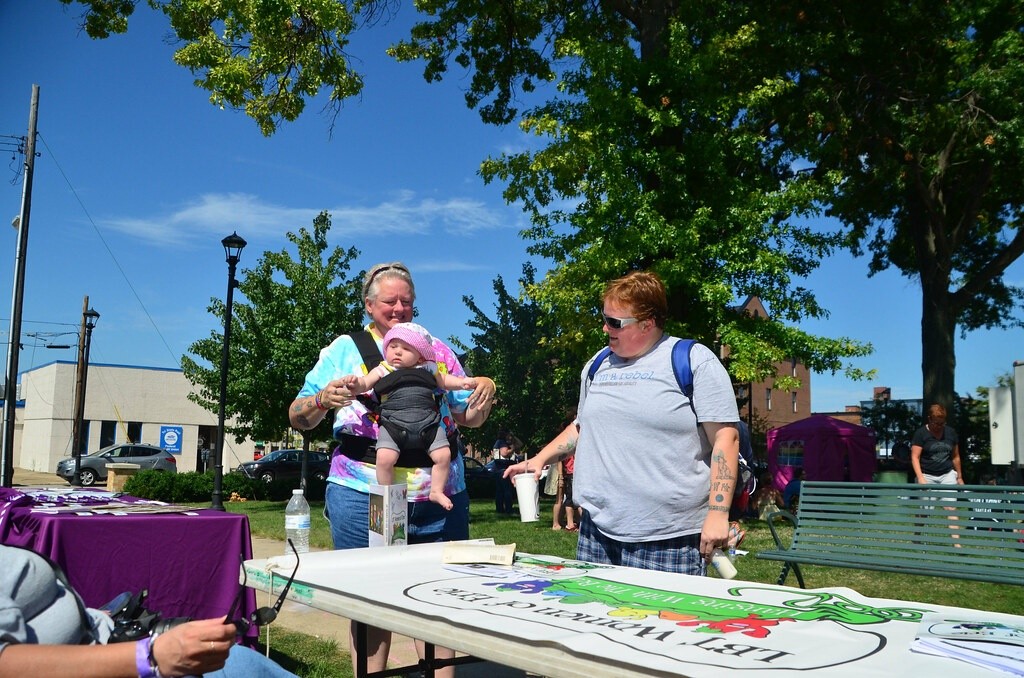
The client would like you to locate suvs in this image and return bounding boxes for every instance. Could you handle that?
[57,442,177,486]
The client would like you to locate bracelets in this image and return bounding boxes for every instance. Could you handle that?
[315,390,329,410]
[148,632,165,678]
[488,378,496,398]
[135,637,155,678]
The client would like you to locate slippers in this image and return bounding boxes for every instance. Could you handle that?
[729,522,739,537]
[736,530,746,549]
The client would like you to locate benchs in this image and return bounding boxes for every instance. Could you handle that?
[747,483,1024,590]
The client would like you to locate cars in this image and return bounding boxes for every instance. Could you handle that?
[463,457,486,498]
[236,450,330,482]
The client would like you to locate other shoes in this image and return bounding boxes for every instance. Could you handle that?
[565,525,578,532]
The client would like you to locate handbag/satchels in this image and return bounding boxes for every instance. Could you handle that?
[544,462,557,495]
[758,500,782,520]
[495,445,517,479]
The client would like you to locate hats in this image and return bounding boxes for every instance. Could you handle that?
[736,395,749,410]
[382,322,435,360]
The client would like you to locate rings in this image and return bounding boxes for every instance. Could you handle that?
[210,640,217,654]
[335,388,337,394]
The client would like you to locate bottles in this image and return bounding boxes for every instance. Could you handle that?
[708,546,738,579]
[729,543,736,564]
[285,489,310,559]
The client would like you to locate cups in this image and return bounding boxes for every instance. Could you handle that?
[514,473,540,523]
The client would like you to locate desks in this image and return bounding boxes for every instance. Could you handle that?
[237,538,1024,678]
[0,487,259,648]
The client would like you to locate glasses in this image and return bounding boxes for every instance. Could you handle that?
[223,538,300,636]
[601,310,653,329]
[930,417,947,427]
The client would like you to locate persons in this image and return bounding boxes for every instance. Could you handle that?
[493,431,516,460]
[783,467,808,508]
[286,262,497,678]
[550,454,583,532]
[0,543,303,678]
[908,401,969,553]
[338,321,479,511]
[752,472,784,522]
[502,271,741,577]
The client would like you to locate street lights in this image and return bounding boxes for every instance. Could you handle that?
[882,389,890,460]
[210,230,248,512]
[69,308,100,486]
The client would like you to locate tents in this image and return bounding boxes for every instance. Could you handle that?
[763,415,877,493]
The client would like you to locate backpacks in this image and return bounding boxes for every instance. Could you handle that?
[589,339,752,497]
